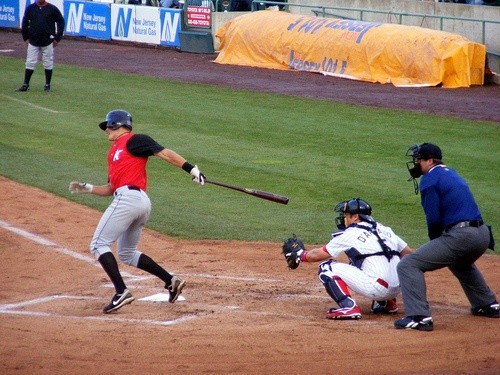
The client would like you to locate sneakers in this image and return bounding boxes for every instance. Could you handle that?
[470,299,500,318]
[324,306,362,320]
[395,316,433,331]
[165,276,186,303]
[44,85,50,93]
[373,300,398,313]
[19,85,27,91]
[102,290,135,313]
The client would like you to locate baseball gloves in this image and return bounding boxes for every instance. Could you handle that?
[283,238,305,270]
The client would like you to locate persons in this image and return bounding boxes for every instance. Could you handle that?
[394,143,500,332]
[283,197,413,320]
[69,110,207,314]
[16,0,65,93]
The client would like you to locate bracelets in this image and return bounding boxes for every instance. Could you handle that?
[182,162,193,174]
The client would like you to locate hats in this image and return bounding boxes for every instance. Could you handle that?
[418,143,444,165]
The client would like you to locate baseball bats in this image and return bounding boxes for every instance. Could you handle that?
[200,179,289,204]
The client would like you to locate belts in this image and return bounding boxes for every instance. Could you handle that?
[115,186,140,196]
[451,220,484,229]
[377,279,388,289]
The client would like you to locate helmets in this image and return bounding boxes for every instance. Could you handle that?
[345,199,376,223]
[98,110,132,132]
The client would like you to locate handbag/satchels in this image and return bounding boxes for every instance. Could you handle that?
[485,225,495,251]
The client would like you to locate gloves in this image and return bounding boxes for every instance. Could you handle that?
[182,163,206,186]
[69,181,94,194]
